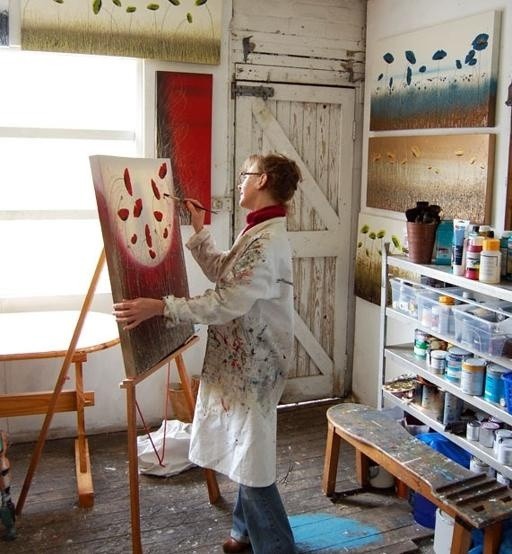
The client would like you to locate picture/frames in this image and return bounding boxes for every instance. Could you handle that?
[351,210,410,312]
[368,8,503,133]
[366,131,498,227]
[19,0,223,67]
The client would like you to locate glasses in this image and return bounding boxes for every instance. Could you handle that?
[239,170,262,183]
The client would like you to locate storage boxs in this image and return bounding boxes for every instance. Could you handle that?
[387,277,424,318]
[415,290,468,338]
[451,303,512,359]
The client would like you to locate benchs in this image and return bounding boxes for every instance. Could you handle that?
[320,401,511,554]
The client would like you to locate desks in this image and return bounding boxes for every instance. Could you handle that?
[0,311,122,510]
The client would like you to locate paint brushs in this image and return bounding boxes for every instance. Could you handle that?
[163,193,218,215]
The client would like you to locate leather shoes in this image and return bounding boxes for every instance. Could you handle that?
[222,534,254,554]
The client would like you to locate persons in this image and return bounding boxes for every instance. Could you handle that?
[112,152,303,554]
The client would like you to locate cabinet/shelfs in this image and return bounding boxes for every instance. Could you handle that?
[376,241,512,494]
